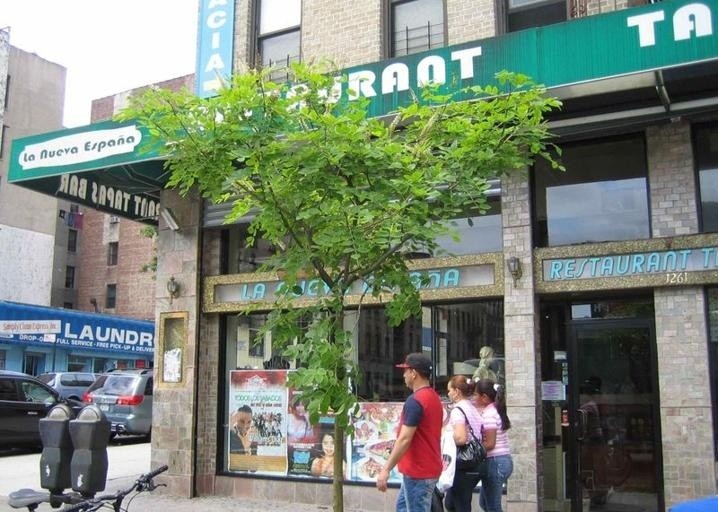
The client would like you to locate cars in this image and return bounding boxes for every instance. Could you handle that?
[0,368,152,448]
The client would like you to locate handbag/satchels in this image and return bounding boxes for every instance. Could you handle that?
[436,425,455,492]
[451,407,486,468]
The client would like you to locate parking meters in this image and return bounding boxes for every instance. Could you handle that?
[38,403,111,500]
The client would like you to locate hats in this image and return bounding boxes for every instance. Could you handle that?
[394,352,434,374]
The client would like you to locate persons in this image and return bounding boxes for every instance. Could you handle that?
[308,430,346,478]
[376,353,445,512]
[228,403,259,474]
[286,394,313,441]
[472,344,501,384]
[473,378,514,511]
[431,376,486,511]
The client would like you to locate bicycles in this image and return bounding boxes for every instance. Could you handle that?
[8,465,169,512]
